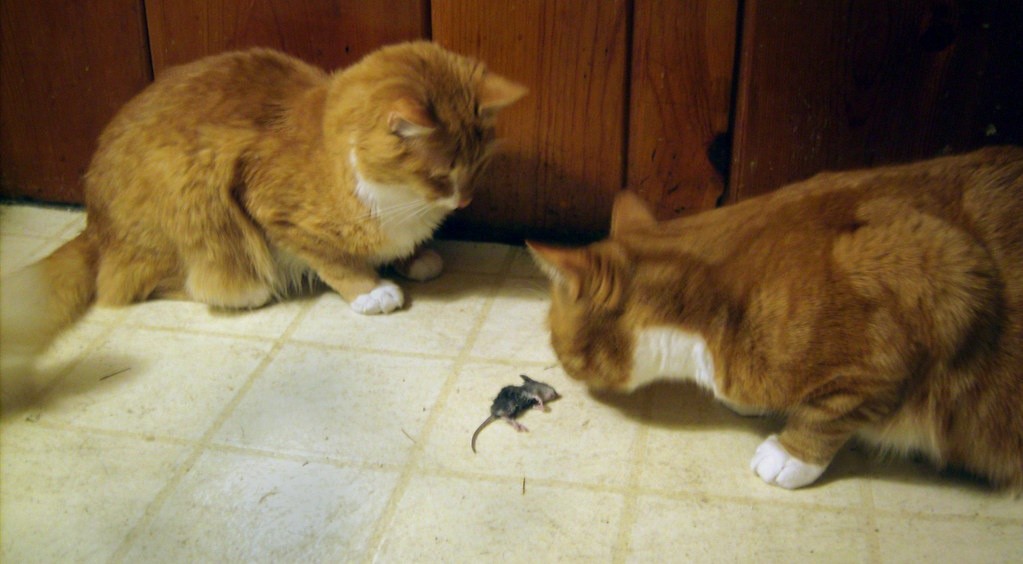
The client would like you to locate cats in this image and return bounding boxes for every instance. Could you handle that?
[525,144,1022,499]
[1,41,527,414]
